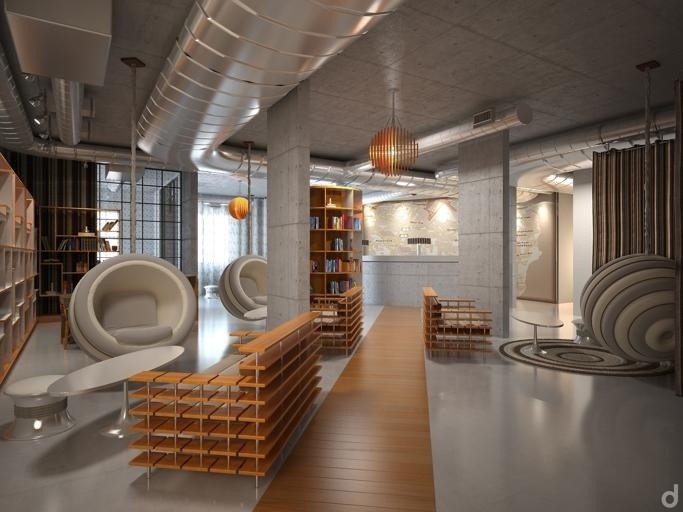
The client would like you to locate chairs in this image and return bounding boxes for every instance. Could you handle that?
[67,256,198,361]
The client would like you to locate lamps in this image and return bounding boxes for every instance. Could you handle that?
[369,89,418,178]
[23,71,51,144]
[228,160,250,220]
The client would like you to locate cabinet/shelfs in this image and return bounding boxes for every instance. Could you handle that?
[0,155,119,383]
[421,286,493,359]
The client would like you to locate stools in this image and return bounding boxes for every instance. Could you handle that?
[1,375,77,443]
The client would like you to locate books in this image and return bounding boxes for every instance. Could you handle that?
[40,220,118,313]
[310,213,361,294]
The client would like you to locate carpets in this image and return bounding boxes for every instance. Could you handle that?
[499,337,674,377]
